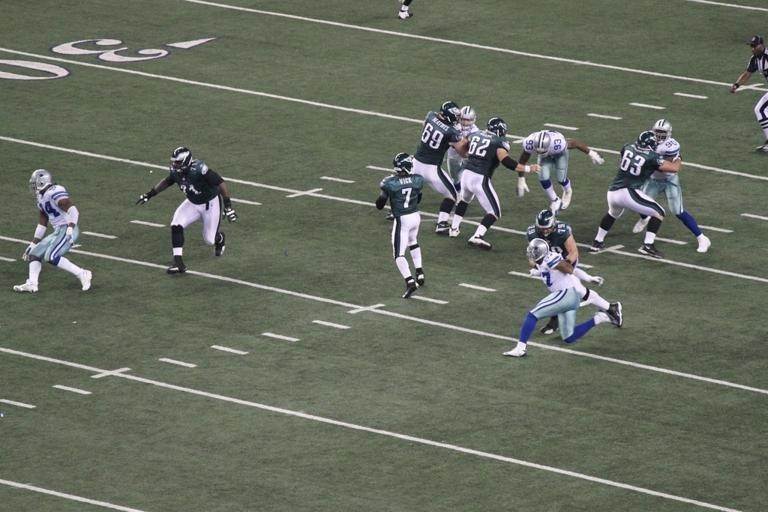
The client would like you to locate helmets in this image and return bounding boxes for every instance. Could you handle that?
[652,119,673,144]
[394,152,415,177]
[531,132,551,155]
[440,101,507,135]
[526,238,549,268]
[634,130,657,152]
[169,147,193,175]
[29,169,52,197]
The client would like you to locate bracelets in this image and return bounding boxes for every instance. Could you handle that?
[734,83,739,88]
[30,242,36,248]
[66,227,73,235]
[223,196,231,208]
[148,189,156,196]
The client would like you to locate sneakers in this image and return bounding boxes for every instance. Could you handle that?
[398,10,414,20]
[79,268,93,291]
[386,209,394,220]
[215,232,226,256]
[503,302,622,357]
[402,282,420,298]
[167,264,186,273]
[548,198,561,216]
[434,220,494,250]
[415,272,424,285]
[13,278,39,293]
[756,143,768,153]
[592,216,711,259]
[560,191,571,209]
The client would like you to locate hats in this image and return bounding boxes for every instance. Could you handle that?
[746,36,765,46]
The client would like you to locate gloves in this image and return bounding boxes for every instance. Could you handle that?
[516,177,530,197]
[457,156,468,170]
[63,226,74,246]
[222,206,238,222]
[587,150,605,166]
[22,241,36,261]
[589,275,604,288]
[528,269,542,279]
[135,191,151,205]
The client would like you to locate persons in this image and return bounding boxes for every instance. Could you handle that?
[13,169,92,293]
[136,147,238,274]
[398,0,413,20]
[729,34,768,153]
